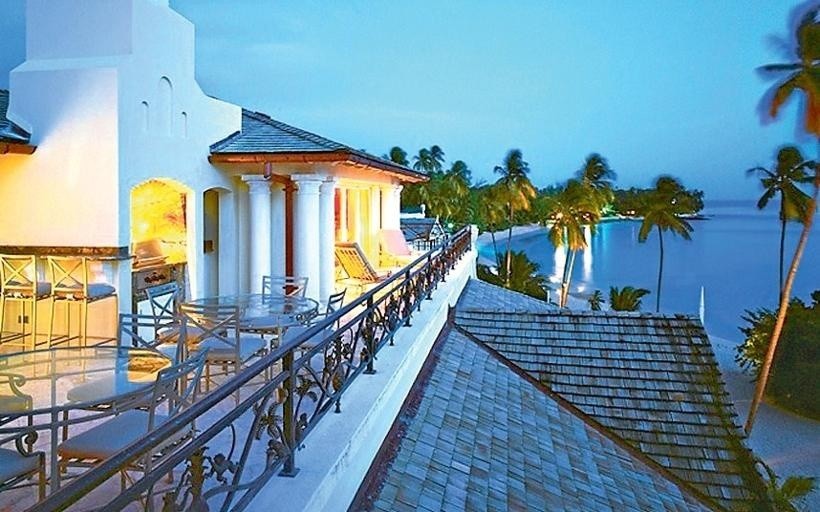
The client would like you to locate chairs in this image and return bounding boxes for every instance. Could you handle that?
[268,286,347,387]
[58,344,212,507]
[263,273,310,298]
[178,301,270,418]
[114,312,188,420]
[336,227,433,309]
[0,355,37,482]
[0,420,48,509]
[144,279,229,394]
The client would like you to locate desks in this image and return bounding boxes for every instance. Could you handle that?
[180,294,320,386]
[1,347,176,492]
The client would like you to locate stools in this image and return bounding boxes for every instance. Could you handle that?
[0,252,71,352]
[46,254,119,351]
